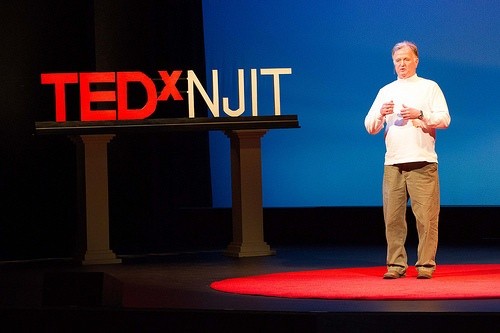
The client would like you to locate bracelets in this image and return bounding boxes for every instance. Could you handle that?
[419,110,423,119]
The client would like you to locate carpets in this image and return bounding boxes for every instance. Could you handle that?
[210,263,500,301]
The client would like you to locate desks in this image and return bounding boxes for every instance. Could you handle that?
[34,114,303,266]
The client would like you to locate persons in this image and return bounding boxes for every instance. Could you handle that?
[364,42,452,279]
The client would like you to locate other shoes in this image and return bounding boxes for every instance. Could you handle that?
[384,271,406,279]
[417,270,432,278]
